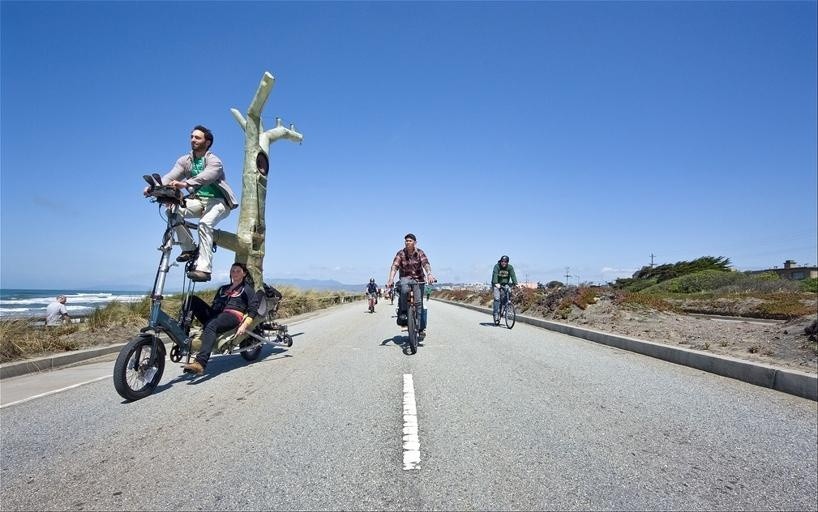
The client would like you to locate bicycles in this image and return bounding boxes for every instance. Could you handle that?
[385,287,397,305]
[385,280,438,354]
[113,172,294,400]
[424,285,435,301]
[366,291,380,313]
[492,282,518,329]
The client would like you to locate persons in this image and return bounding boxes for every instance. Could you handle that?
[177,263,258,377]
[364,276,433,308]
[143,124,234,283]
[44,293,72,328]
[490,254,517,329]
[384,233,434,341]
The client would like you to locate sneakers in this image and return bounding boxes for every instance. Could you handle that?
[187,271,210,281]
[184,362,203,373]
[176,251,194,262]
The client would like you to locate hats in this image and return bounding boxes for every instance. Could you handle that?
[405,234,416,241]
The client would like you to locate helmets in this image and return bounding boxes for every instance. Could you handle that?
[501,256,509,261]
